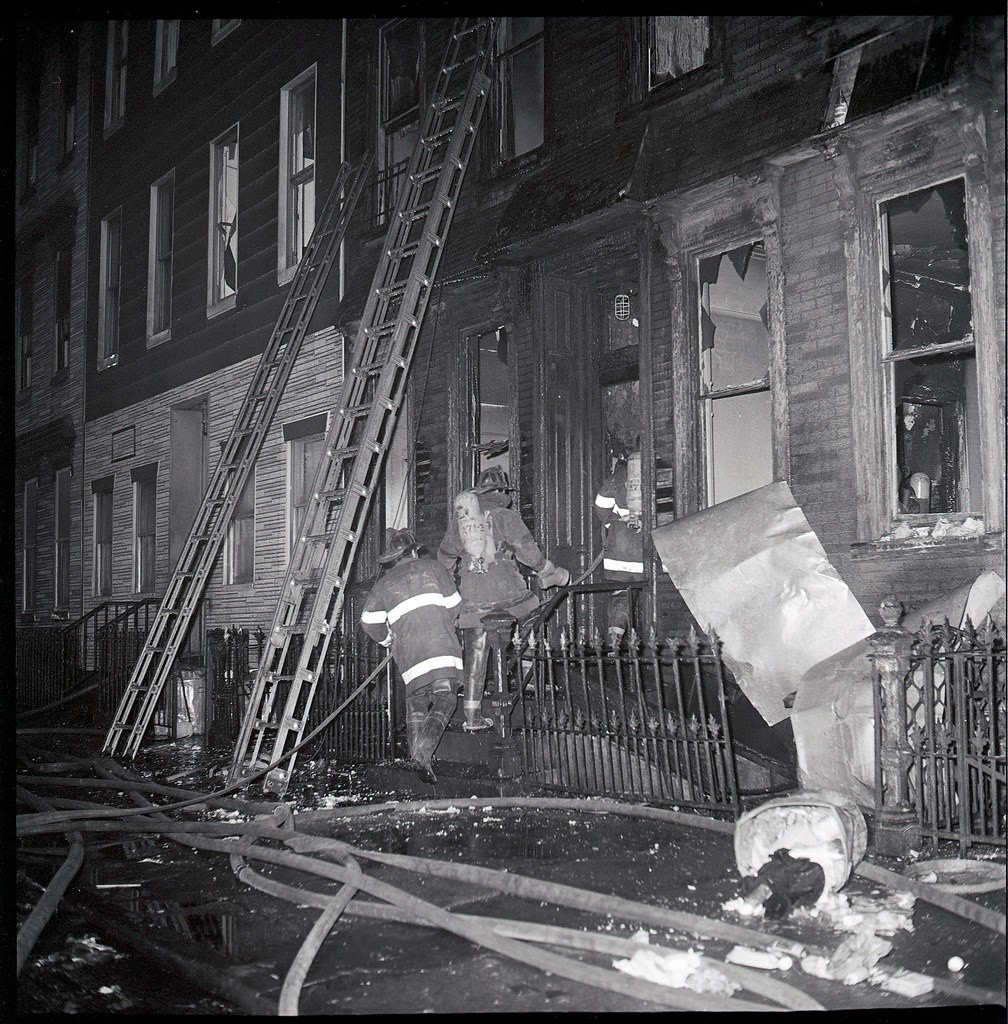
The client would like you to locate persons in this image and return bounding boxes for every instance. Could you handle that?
[437,467,557,728]
[594,435,644,657]
[361,534,465,785]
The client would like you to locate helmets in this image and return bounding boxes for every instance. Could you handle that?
[469,467,515,494]
[377,533,424,562]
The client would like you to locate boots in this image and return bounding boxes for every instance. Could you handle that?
[519,614,557,693]
[406,697,428,758]
[407,692,457,785]
[608,588,631,659]
[463,627,494,733]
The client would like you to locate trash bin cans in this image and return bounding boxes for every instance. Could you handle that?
[154,670,212,738]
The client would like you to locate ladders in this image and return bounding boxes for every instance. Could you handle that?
[230,17,511,796]
[101,147,375,759]
